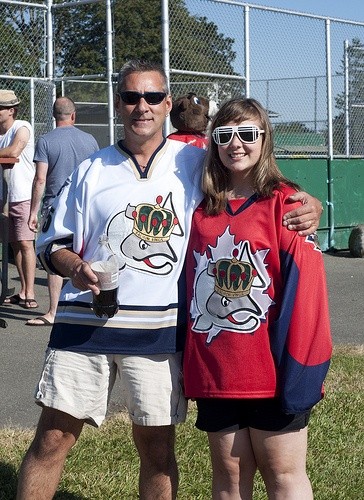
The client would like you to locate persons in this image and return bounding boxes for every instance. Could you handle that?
[175,96,333,500]
[16,60,323,500]
[26,97,100,327]
[0,89,39,308]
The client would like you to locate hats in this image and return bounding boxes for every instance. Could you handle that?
[0,90,21,106]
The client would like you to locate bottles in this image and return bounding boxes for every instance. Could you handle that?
[88,233,119,319]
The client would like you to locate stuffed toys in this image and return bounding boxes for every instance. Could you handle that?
[166,92,221,151]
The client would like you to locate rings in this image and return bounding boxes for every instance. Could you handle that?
[309,221,313,227]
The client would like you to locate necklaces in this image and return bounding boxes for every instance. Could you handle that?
[139,163,146,168]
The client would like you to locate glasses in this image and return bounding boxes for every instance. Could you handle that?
[212,125,265,146]
[117,91,167,105]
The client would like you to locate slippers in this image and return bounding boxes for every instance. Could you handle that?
[27,316,55,326]
[3,293,39,308]
